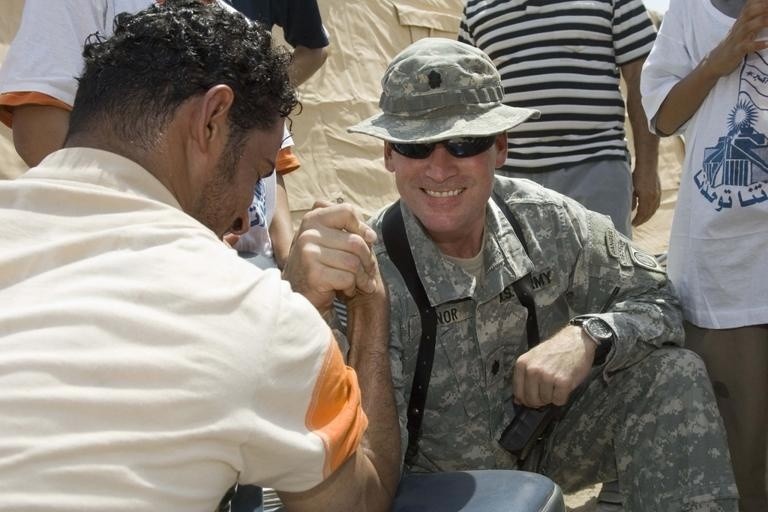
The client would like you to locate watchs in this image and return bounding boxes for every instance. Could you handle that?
[566,315,615,368]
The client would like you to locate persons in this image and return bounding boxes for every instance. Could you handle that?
[2,2,305,512]
[277,35,743,510]
[160,0,333,109]
[1,2,404,510]
[452,2,667,505]
[635,1,768,509]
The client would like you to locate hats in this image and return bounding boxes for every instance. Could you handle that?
[342,34,545,150]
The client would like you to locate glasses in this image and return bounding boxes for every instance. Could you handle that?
[382,131,502,159]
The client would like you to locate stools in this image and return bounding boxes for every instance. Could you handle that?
[390,470,565,512]
